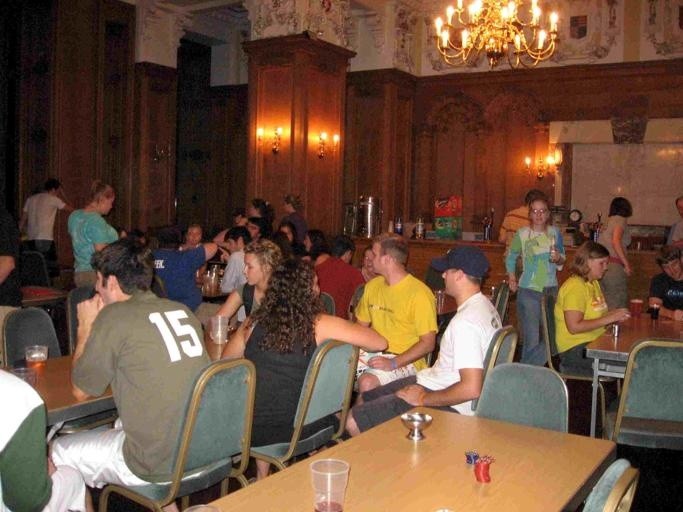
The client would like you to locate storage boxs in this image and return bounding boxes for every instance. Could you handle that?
[462,232,484,241]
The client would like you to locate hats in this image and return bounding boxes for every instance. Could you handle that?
[432,246,490,278]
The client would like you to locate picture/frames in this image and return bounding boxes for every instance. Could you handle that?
[628,224,669,243]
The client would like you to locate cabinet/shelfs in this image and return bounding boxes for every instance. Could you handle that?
[351,239,666,340]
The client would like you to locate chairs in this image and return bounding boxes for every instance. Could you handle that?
[475,364,568,432]
[2,309,115,434]
[470,325,520,411]
[100,358,257,512]
[222,338,359,497]
[65,287,99,354]
[604,339,683,454]
[492,283,510,325]
[18,251,49,289]
[540,287,621,432]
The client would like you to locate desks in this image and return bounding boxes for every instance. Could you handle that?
[21,287,69,323]
[195,406,618,511]
[6,336,248,445]
[586,306,683,437]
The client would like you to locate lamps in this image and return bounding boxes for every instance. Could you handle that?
[428,0,567,68]
[525,156,554,180]
[312,128,340,158]
[257,127,282,153]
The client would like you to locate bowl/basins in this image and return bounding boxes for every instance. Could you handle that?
[399,412,432,441]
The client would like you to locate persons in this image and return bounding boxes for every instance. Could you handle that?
[316,236,368,322]
[22,177,76,276]
[68,180,127,289]
[151,228,237,328]
[246,217,272,240]
[237,200,277,238]
[275,218,310,260]
[648,247,683,322]
[595,197,633,311]
[211,239,283,339]
[667,196,683,251]
[179,226,205,253]
[341,247,502,436]
[0,206,24,369]
[0,369,93,512]
[221,258,388,485]
[351,233,438,406]
[357,245,382,283]
[116,225,154,250]
[554,239,633,426]
[504,197,565,366]
[280,193,308,242]
[301,229,329,266]
[216,226,247,292]
[498,190,549,253]
[45,238,213,512]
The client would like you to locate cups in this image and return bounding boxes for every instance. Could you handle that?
[24,345,48,377]
[9,367,36,392]
[433,290,445,312]
[308,458,350,511]
[629,299,644,318]
[210,314,229,344]
[649,304,660,320]
[200,271,218,295]
[211,265,220,278]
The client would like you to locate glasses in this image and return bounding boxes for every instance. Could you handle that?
[533,209,545,213]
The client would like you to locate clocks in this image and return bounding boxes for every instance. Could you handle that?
[569,209,581,223]
